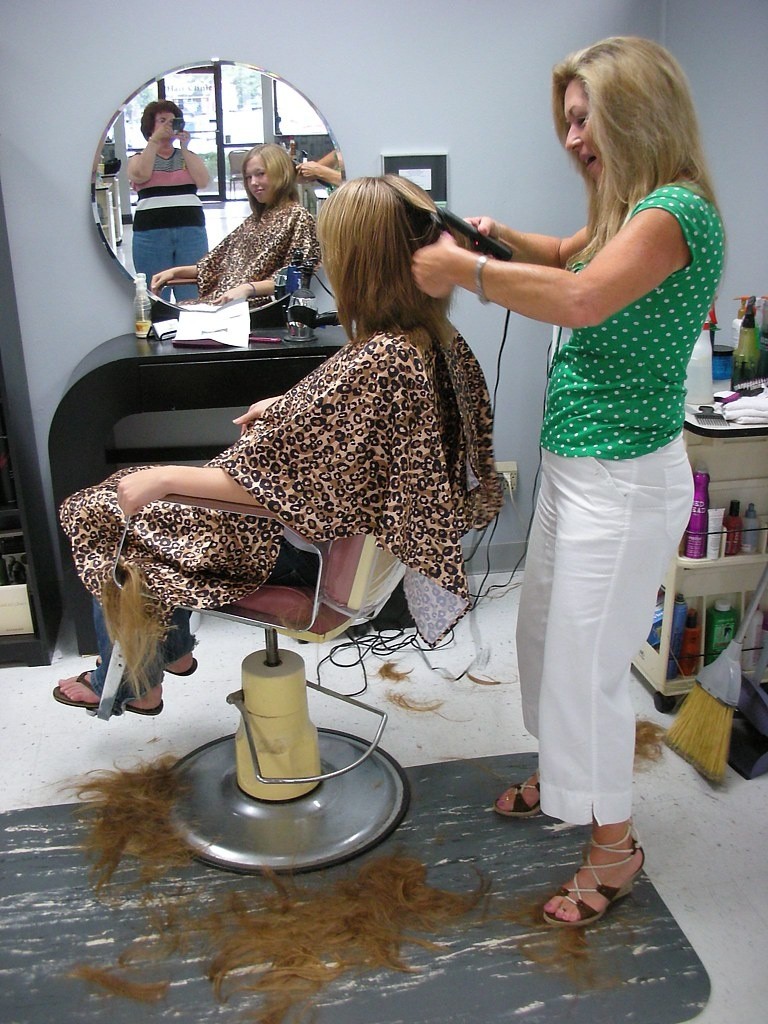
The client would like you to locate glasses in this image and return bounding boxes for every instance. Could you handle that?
[154,118,165,124]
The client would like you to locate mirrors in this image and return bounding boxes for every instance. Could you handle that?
[90,57,346,314]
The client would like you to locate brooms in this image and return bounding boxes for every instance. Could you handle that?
[660,563,768,783]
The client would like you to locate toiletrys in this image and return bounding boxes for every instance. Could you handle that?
[722,499,743,556]
[683,459,712,559]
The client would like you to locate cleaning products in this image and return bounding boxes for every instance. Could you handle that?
[705,598,737,665]
[740,602,765,671]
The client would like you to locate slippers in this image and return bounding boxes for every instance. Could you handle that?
[96,653,198,676]
[54,669,163,716]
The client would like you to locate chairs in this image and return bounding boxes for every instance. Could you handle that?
[87,480,410,878]
[228,149,250,193]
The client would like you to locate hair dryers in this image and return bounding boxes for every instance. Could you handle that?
[272,263,304,301]
[285,287,342,341]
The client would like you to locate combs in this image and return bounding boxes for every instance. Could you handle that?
[720,378,768,401]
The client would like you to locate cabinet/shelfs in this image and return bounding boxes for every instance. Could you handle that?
[630,402,768,714]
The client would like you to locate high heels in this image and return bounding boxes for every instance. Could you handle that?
[543,816,645,928]
[494,769,542,817]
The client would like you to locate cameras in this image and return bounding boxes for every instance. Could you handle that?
[172,117,185,135]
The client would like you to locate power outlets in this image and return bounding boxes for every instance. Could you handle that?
[495,461,517,492]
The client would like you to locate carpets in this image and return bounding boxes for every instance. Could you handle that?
[0,752,712,1024]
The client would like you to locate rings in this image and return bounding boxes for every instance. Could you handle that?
[300,170,302,175]
[166,129,169,131]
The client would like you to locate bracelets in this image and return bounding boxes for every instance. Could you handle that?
[474,257,489,304]
[249,282,258,295]
[148,135,159,143]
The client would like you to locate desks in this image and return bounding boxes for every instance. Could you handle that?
[100,171,123,247]
[48,324,351,658]
[95,182,118,255]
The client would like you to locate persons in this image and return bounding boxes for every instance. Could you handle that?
[128,100,209,301]
[412,36,723,928]
[287,140,304,168]
[151,144,321,309]
[50,174,507,715]
[295,149,343,191]
